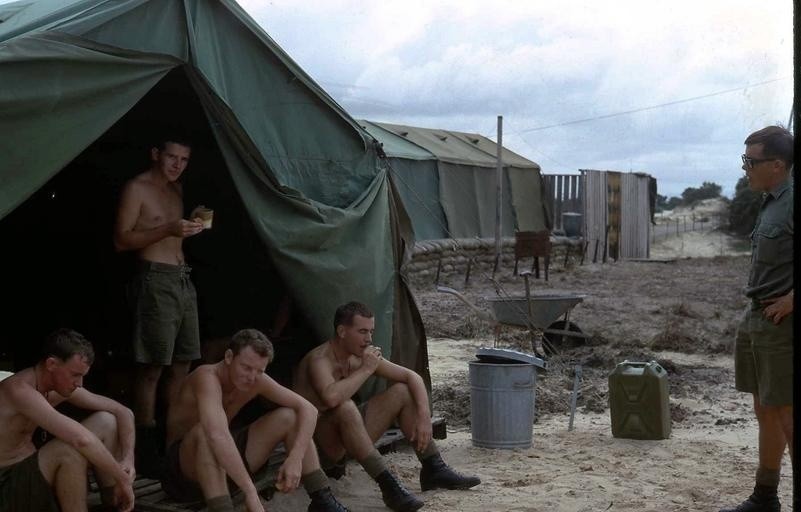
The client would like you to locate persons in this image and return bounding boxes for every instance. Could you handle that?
[0,331,136,512]
[721,126,794,512]
[113,135,203,420]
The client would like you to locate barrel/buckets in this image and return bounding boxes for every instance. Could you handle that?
[607,359,672,439]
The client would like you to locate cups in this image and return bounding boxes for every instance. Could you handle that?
[195,208,215,230]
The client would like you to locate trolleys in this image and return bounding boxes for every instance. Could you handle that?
[437,285,593,362]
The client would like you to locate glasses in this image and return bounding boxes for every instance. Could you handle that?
[742,154,775,169]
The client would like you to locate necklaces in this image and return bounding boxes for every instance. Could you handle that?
[33,366,48,443]
[329,339,351,380]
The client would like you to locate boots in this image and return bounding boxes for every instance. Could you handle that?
[374,469,424,512]
[307,486,351,512]
[420,453,481,491]
[718,483,781,512]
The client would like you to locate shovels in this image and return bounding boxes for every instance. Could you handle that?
[520,269,548,361]
